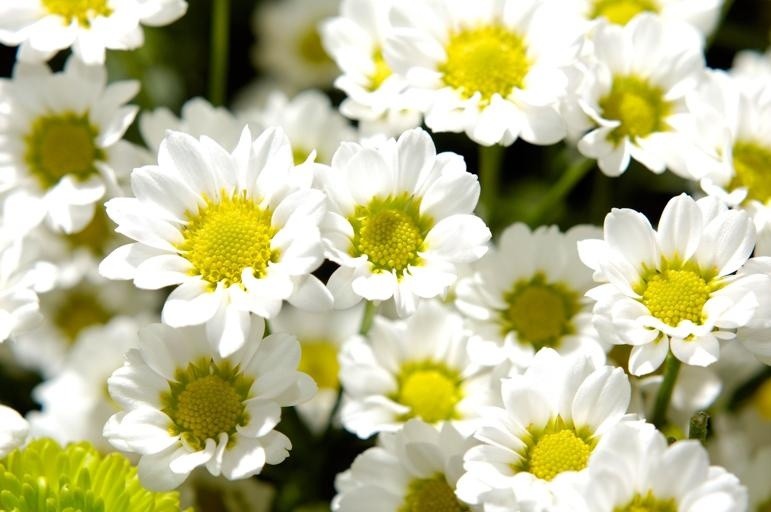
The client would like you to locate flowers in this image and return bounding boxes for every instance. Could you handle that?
[0,0,771,512]
